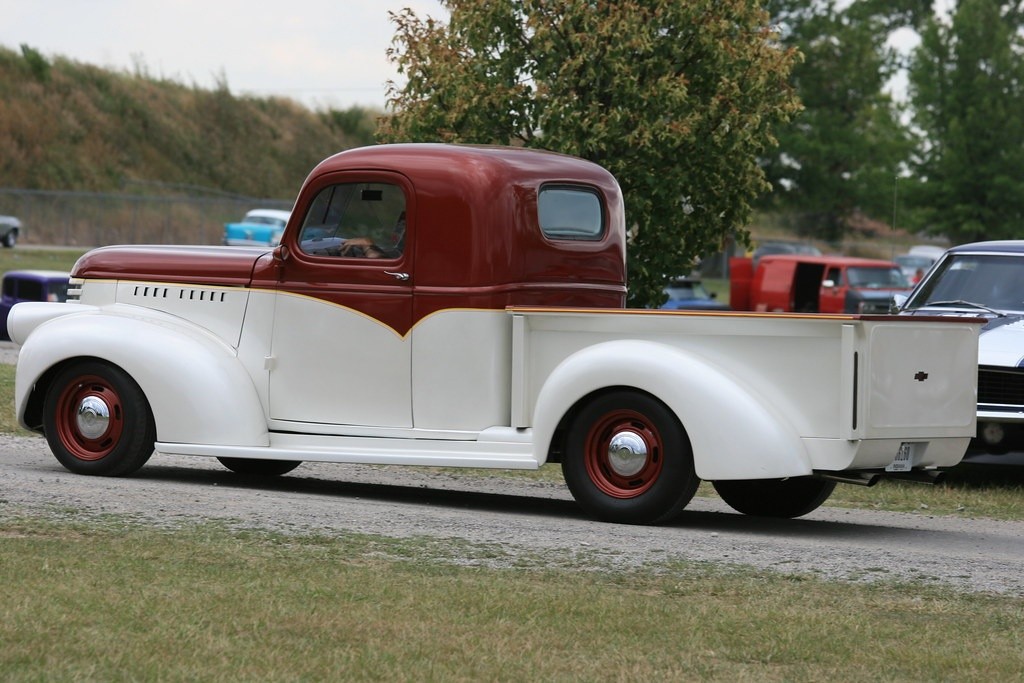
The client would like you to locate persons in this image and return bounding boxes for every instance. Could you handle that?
[912,268,922,284]
[340,238,388,260]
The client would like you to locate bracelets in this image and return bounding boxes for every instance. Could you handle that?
[362,244,378,254]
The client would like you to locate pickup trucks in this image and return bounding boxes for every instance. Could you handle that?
[0,142,989,528]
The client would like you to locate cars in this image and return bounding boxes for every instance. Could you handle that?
[0,215,21,248]
[651,234,1024,459]
[221,206,324,246]
[0,270,73,341]
